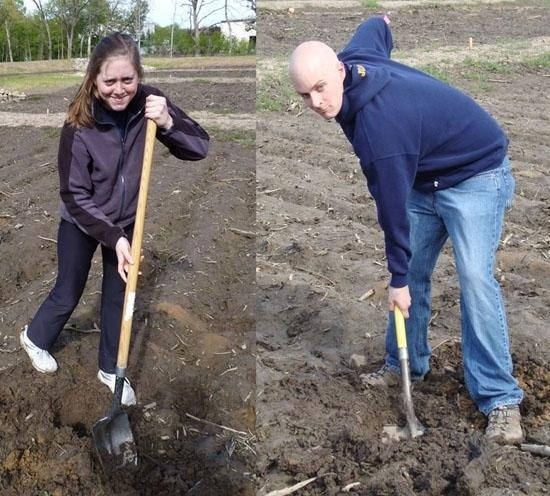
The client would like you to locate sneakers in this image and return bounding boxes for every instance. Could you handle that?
[359,365,424,386]
[20,324,58,373]
[486,404,523,443]
[97,369,137,407]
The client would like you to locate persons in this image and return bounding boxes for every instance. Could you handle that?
[289,15,525,445]
[18,29,210,407]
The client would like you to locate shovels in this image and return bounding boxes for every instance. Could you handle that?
[91,118,156,474]
[375,305,428,442]
